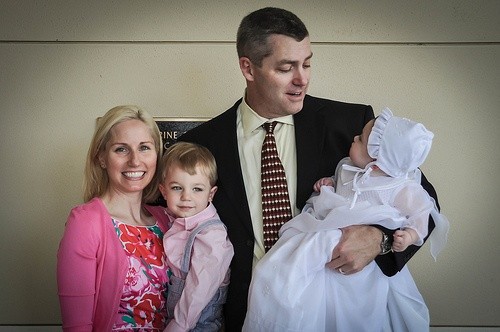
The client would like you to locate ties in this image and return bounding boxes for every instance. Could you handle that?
[259,121,293,253]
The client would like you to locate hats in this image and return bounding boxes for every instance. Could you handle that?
[367,108,435,177]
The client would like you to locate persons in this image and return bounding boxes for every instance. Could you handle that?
[56,104,174,332]
[156,142,235,332]
[178,7,441,332]
[241,108,449,332]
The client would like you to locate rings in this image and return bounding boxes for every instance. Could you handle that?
[339,267,344,273]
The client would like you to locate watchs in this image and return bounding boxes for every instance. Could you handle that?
[381,234,391,252]
